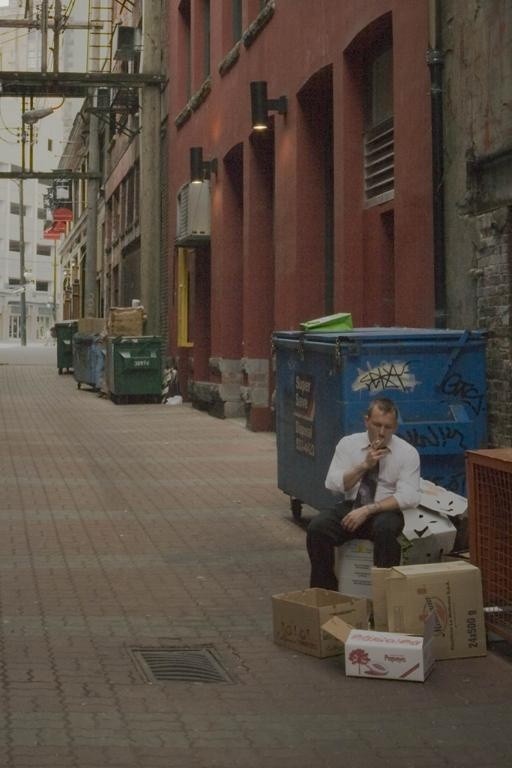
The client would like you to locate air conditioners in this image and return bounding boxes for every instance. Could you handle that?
[174,179,209,247]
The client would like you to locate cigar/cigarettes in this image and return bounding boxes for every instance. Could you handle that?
[375,439,381,444]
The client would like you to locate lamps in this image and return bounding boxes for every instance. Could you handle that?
[190,143,217,185]
[249,77,287,133]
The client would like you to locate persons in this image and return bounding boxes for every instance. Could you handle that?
[304,395,422,592]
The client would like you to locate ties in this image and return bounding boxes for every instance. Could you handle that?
[353,459,380,508]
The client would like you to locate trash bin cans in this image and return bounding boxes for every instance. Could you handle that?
[272,311,489,520]
[55,320,163,404]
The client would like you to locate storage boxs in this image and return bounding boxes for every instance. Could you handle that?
[78,306,146,338]
[272,587,370,658]
[322,616,436,682]
[371,560,487,660]
